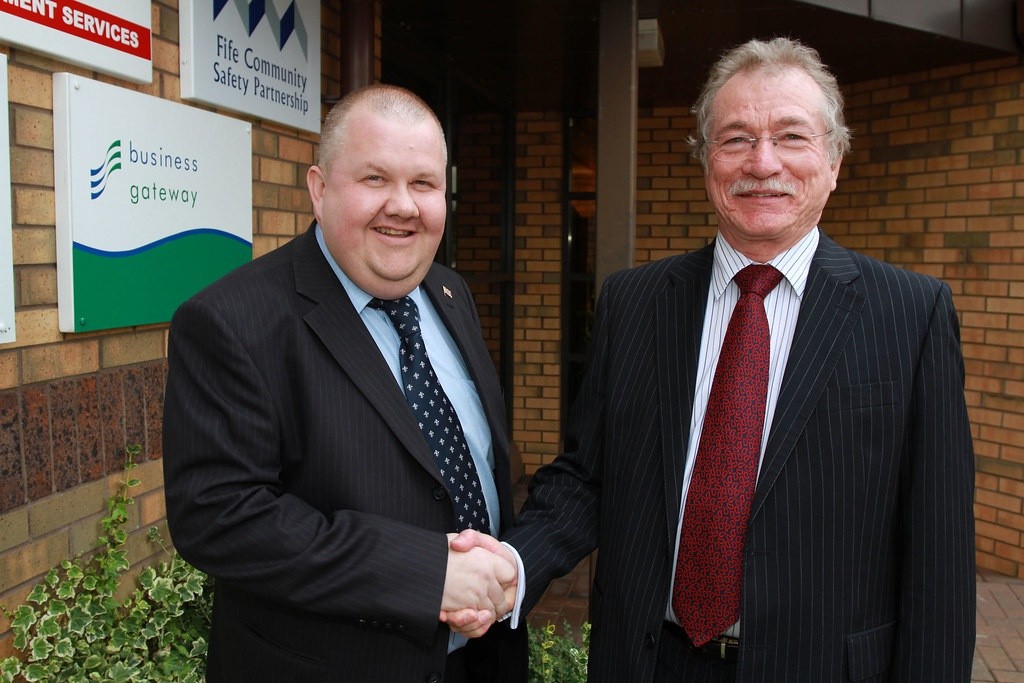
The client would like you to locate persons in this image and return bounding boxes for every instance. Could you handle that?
[161,85,530,683]
[443,37,976,683]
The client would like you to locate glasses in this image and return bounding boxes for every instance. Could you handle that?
[704,128,838,160]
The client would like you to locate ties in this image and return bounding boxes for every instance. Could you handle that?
[670,261,785,647]
[365,299,492,554]
